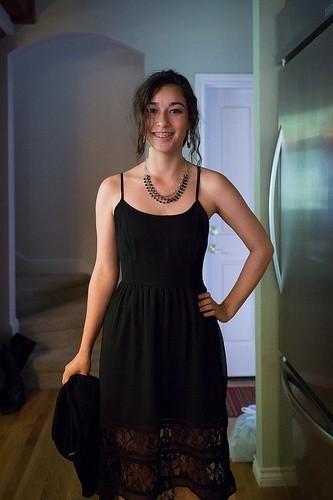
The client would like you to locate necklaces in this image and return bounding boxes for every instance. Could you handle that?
[141,158,190,205]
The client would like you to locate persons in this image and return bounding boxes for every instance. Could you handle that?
[50,69,276,500]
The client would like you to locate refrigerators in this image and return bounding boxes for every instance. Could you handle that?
[268,0,333,500]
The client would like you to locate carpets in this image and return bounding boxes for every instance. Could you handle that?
[225,384,256,417]
